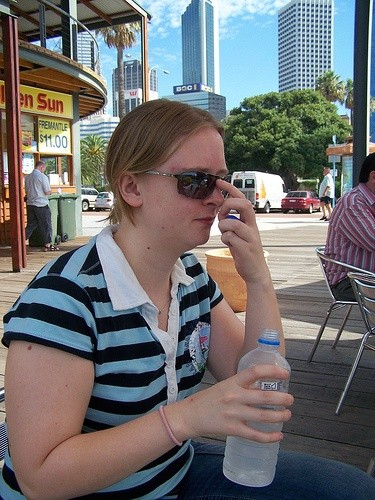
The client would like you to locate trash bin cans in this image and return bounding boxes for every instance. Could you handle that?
[47,199,59,245]
[60,195,78,242]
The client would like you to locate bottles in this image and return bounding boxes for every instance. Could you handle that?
[223,327,291,487]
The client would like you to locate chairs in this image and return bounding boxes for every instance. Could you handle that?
[309,246,375,360]
[335,271,375,417]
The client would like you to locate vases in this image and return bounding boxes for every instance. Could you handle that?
[205,247,268,314]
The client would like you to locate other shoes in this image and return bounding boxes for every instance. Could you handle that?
[320,216,326,220]
[325,218,330,221]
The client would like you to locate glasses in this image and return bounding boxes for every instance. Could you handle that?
[144,166,233,201]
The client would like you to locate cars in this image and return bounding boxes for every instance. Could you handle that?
[81,188,99,212]
[94,192,114,211]
[281,190,322,215]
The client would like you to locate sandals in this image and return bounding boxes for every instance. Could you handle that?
[43,243,59,251]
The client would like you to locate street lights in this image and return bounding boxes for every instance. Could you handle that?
[148,66,170,99]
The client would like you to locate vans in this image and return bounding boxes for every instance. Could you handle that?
[231,171,290,215]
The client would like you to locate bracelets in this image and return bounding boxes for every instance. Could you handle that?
[158,405,183,446]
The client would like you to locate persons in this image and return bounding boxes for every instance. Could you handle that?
[0,99,375,500]
